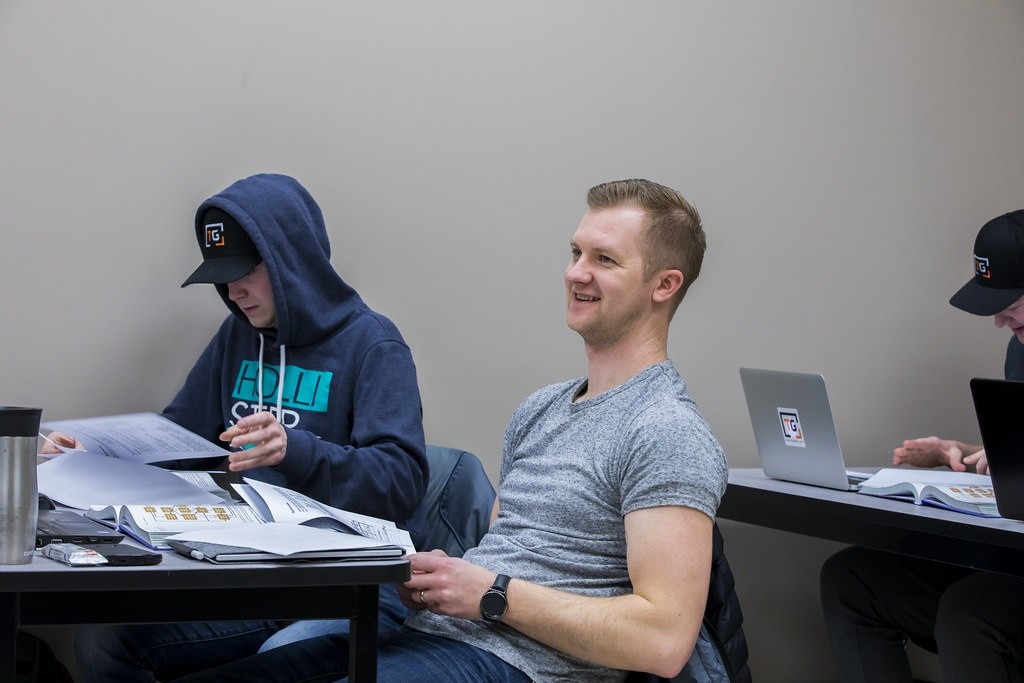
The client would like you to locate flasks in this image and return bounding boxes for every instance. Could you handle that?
[0,406,43,565]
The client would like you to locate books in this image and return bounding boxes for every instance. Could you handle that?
[858,468,1001,519]
[84,505,407,564]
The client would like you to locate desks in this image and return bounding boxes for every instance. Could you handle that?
[700,453,1024,683]
[0,450,419,683]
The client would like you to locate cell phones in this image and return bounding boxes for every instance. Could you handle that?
[82,543,162,567]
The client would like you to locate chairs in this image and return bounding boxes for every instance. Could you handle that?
[374,438,502,549]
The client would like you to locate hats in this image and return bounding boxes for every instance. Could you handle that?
[949,209,1024,316]
[181,207,263,288]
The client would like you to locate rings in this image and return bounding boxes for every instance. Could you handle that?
[420,591,424,602]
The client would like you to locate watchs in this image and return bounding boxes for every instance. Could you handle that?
[480,574,512,622]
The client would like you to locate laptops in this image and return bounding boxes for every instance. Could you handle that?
[970,378,1024,520]
[35,510,126,550]
[739,368,876,491]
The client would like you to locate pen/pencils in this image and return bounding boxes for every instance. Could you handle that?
[167,542,204,561]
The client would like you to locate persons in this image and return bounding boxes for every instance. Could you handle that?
[818,209,1024,683]
[42,173,432,683]
[254,178,728,683]
